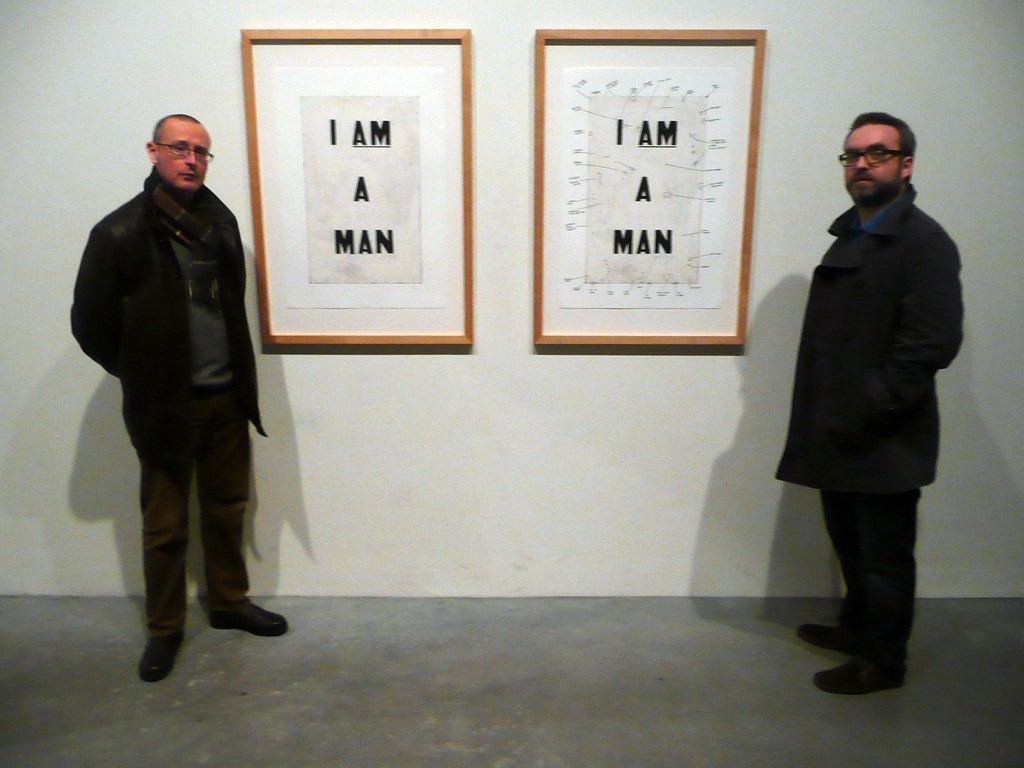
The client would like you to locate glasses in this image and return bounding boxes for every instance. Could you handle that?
[155,142,214,162]
[839,149,909,167]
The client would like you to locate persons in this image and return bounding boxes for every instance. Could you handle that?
[70,114,288,683]
[776,113,964,694]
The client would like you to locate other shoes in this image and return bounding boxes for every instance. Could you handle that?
[814,655,905,693]
[798,622,854,653]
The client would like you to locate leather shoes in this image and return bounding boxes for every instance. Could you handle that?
[210,604,288,637]
[139,631,183,682]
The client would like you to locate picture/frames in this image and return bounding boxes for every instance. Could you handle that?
[533,29,766,345]
[241,30,473,344]
